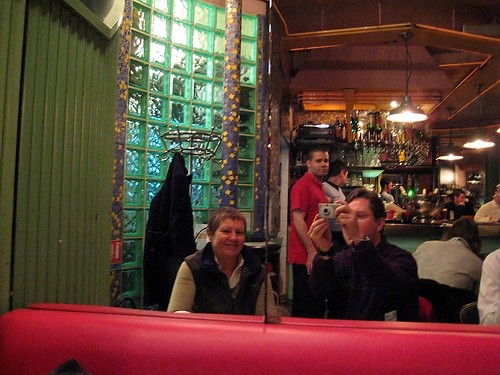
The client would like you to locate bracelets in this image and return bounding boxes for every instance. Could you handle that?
[317,243,335,258]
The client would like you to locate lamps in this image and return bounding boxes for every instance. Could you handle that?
[462,83,496,149]
[385,31,429,123]
[467,172,482,184]
[436,107,464,161]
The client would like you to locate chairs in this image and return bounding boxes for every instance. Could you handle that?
[415,279,477,324]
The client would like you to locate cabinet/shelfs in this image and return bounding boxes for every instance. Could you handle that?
[288,135,439,196]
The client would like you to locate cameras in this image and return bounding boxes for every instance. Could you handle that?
[318,203,343,231]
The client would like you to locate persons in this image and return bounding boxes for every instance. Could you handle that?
[305,188,419,322]
[477,248,500,326]
[379,179,500,223]
[322,159,348,258]
[166,206,276,316]
[288,146,331,318]
[412,217,484,291]
